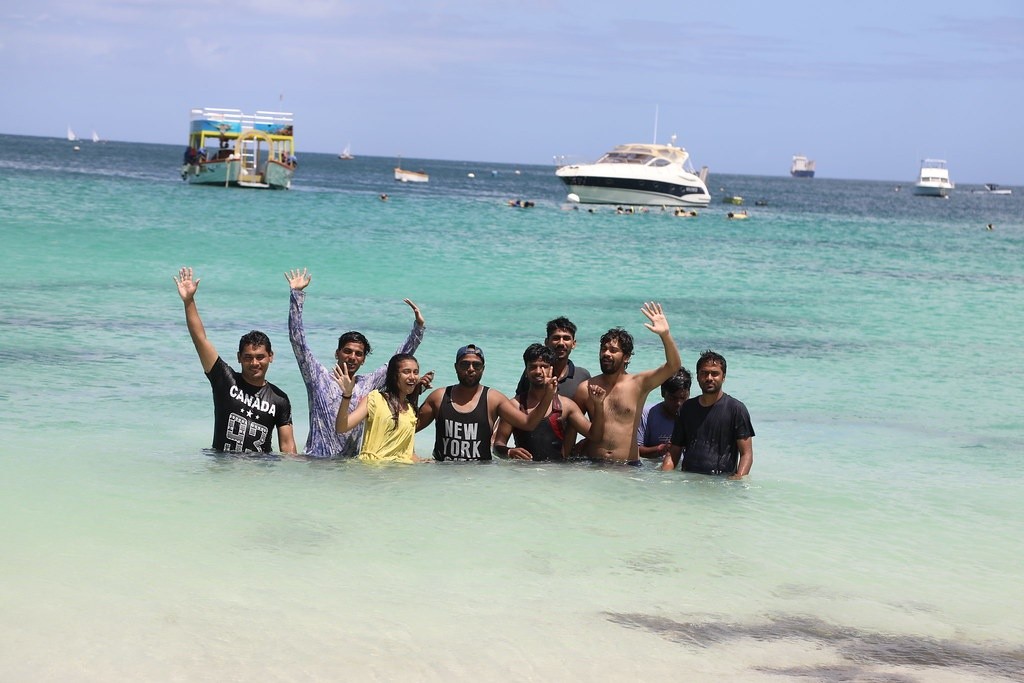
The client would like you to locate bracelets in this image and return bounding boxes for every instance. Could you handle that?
[342,394,351,399]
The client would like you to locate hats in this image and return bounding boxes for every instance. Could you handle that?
[456,344,484,362]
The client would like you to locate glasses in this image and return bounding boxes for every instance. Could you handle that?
[457,362,483,370]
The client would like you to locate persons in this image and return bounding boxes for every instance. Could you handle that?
[330,353,421,460]
[494,318,592,437]
[171,265,296,454]
[183,147,207,164]
[663,350,756,476]
[493,343,607,461]
[564,301,681,461]
[282,153,297,167]
[636,366,692,458]
[212,143,229,160]
[285,267,426,457]
[510,200,534,208]
[407,344,558,461]
[675,209,696,216]
[617,208,632,214]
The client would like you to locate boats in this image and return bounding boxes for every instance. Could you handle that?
[722,195,744,206]
[790,155,817,177]
[181,108,298,190]
[393,167,430,185]
[554,105,713,207]
[972,183,1013,195]
[912,158,955,196]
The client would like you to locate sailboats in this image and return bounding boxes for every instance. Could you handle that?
[339,141,355,160]
